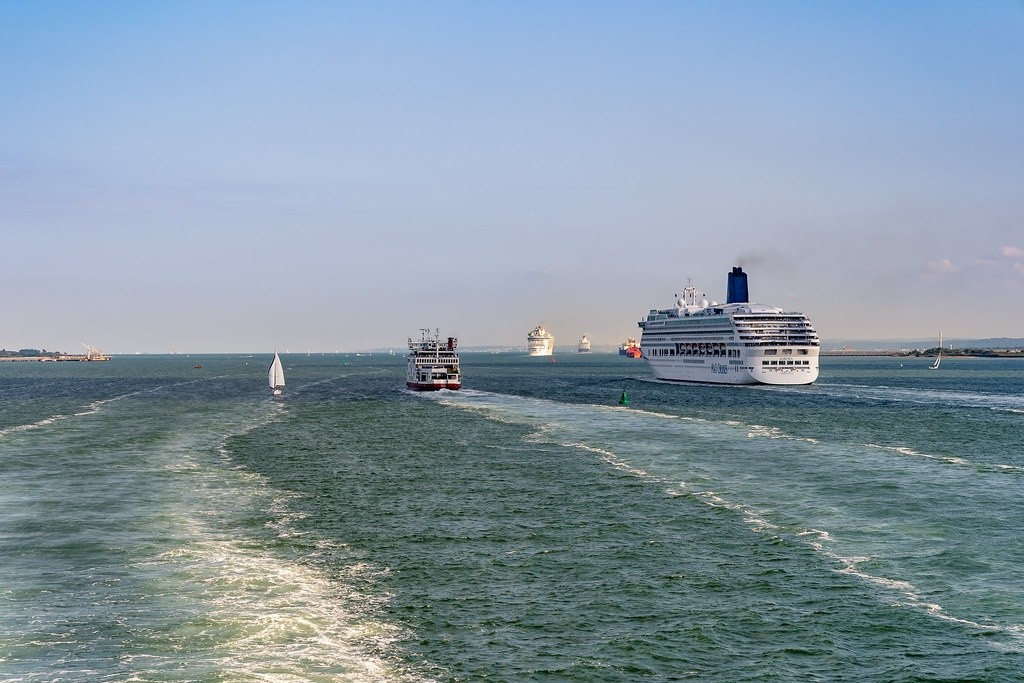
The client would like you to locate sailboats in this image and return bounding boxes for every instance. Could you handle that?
[928,349,941,370]
[268,349,286,395]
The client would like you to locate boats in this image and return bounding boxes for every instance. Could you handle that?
[405,327,461,392]
[527,326,556,357]
[619,338,641,354]
[626,347,642,359]
[577,336,591,352]
[638,275,822,386]
